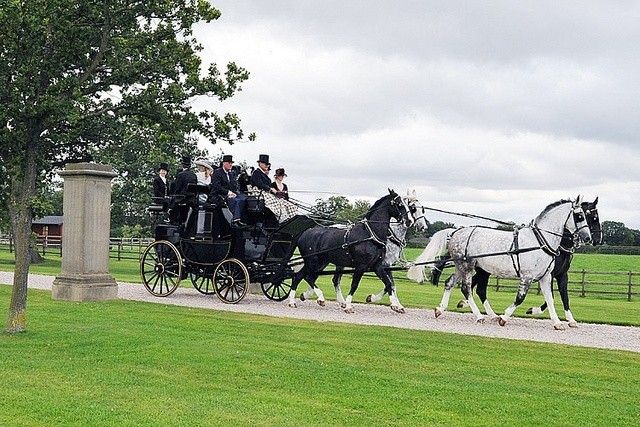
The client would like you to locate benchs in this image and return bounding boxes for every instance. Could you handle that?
[147,184,271,235]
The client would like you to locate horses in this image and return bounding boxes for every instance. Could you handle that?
[288,187,415,314]
[406,191,592,330]
[299,186,428,311]
[430,195,604,327]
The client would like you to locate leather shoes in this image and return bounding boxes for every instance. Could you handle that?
[231,220,247,228]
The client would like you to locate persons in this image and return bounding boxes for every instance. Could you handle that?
[153,163,170,223]
[265,163,271,175]
[250,154,272,192]
[171,169,184,224]
[213,155,250,226]
[170,156,198,239]
[194,161,214,234]
[237,172,251,195]
[269,168,290,201]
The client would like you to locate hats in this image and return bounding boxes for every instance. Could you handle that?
[257,155,270,165]
[222,155,234,163]
[158,163,169,171]
[181,156,191,165]
[194,159,213,171]
[274,169,287,177]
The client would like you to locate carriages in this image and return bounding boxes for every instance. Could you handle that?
[139,187,600,324]
